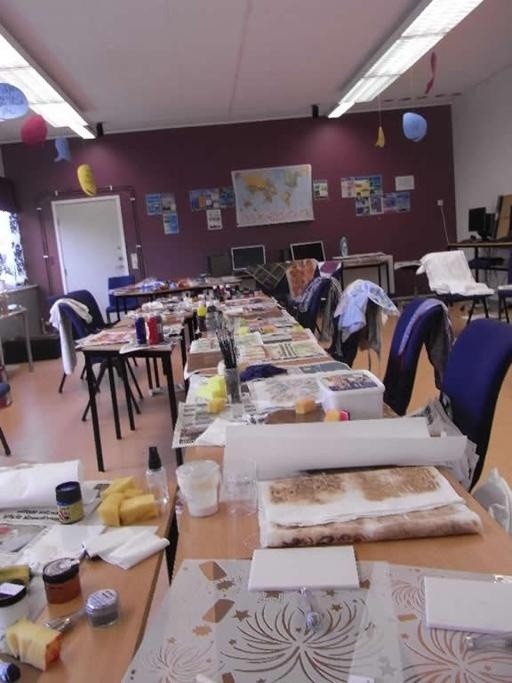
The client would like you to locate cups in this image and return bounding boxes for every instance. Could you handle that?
[177,459,221,519]
[223,348,239,370]
[222,366,244,418]
[0,578,28,654]
[223,458,258,519]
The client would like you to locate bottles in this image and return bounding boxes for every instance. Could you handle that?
[146,444,168,504]
[339,234,348,255]
[175,282,254,331]
[86,588,119,627]
[134,310,164,344]
[1,292,23,315]
[55,481,86,526]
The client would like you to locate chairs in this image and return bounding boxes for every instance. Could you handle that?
[57,275,241,472]
[1,304,34,372]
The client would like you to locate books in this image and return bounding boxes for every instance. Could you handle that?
[248,546,360,591]
[11,524,108,576]
[424,576,512,634]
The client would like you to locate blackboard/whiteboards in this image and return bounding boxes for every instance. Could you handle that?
[231,164,315,228]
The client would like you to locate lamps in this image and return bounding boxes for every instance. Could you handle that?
[69,125,98,140]
[338,0,487,103]
[326,103,355,120]
[0,25,89,128]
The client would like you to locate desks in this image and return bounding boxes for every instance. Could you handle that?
[167,239,512,683]
[0,472,178,683]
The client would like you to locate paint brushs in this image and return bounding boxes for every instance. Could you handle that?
[216,322,240,404]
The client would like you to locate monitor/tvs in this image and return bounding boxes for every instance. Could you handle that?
[289,240,327,263]
[232,244,266,271]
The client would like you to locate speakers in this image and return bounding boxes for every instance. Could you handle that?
[97,122,102,137]
[312,104,319,118]
